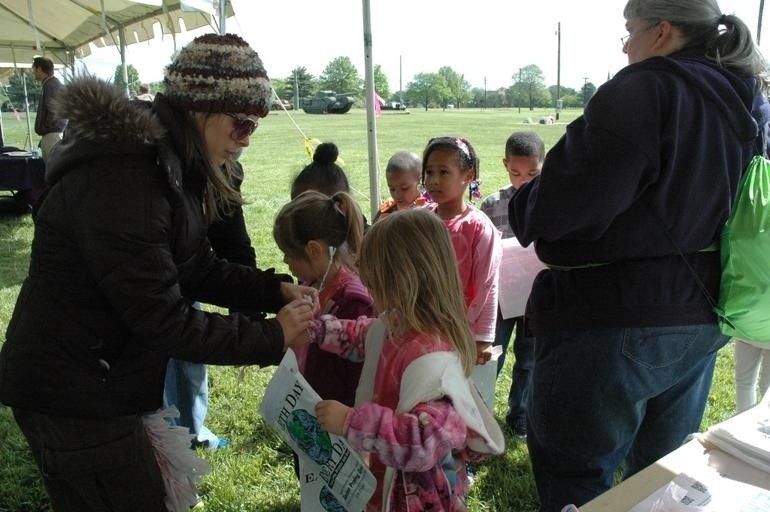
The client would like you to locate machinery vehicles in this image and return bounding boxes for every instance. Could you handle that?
[301,88,357,114]
[271,98,294,112]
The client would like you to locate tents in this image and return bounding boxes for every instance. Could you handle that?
[1,0,369,201]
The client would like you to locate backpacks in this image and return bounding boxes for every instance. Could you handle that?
[643,148,770,344]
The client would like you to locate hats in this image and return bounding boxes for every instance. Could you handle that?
[164,34,273,117]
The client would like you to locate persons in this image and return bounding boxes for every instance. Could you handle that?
[273,129,546,512]
[129,90,137,98]
[163,160,269,455]
[509,2,769,512]
[32,56,67,163]
[399,98,404,110]
[133,83,156,101]
[1,34,322,512]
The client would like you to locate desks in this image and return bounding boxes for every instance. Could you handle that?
[573,385,770,511]
[0,146,46,211]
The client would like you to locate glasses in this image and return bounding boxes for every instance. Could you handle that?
[224,112,258,141]
